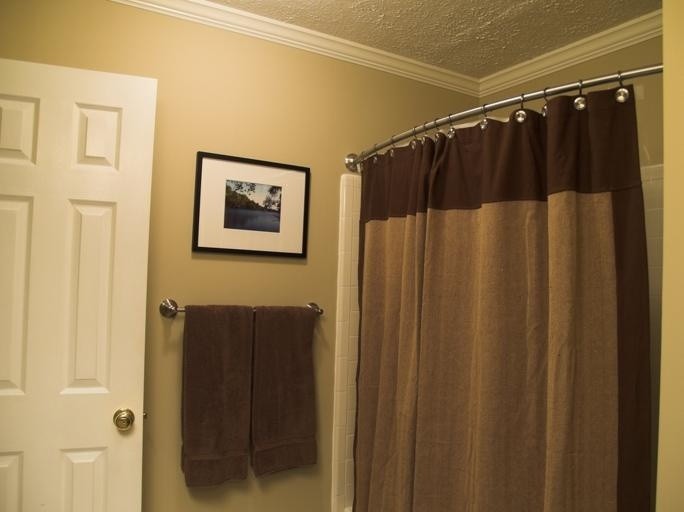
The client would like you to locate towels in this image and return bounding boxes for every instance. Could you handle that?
[181,300,320,485]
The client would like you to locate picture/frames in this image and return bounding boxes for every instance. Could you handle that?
[191,148,310,261]
